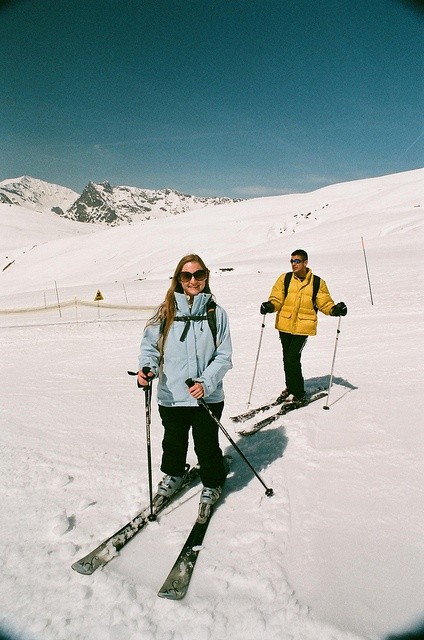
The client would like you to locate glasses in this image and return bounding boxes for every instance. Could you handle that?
[177,270,207,283]
[291,259,303,264]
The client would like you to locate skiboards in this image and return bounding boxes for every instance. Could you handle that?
[230,381,333,437]
[71,455,233,601]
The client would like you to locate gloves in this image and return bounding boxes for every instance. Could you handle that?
[260,302,275,314]
[333,302,347,316]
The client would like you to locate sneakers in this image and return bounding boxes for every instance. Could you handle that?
[157,473,183,496]
[200,486,222,504]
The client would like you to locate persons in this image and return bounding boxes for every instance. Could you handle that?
[136,254,232,505]
[260,249,347,404]
